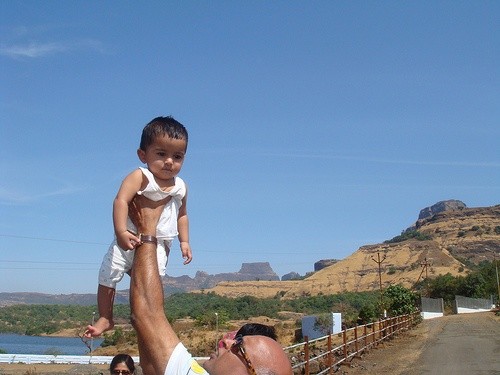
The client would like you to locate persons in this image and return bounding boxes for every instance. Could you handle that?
[83,115,193,338]
[234,323,278,343]
[110,354,136,375]
[126,193,295,375]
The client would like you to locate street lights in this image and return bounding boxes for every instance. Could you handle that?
[215,312,218,350]
[91,312,96,353]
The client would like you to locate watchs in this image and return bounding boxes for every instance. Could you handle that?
[135,234,157,244]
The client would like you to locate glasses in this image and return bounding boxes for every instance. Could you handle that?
[231,334,258,375]
[111,368,134,375]
